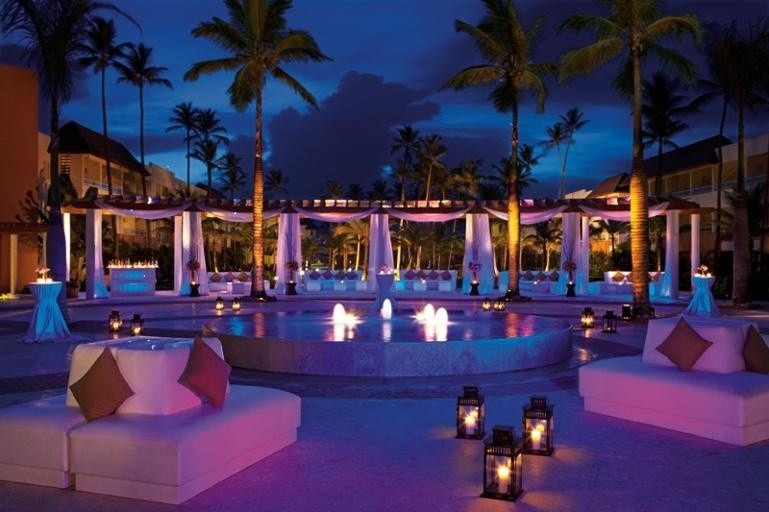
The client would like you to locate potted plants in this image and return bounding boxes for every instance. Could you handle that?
[468,261,482,296]
[563,261,577,297]
[186,258,201,297]
[286,261,299,295]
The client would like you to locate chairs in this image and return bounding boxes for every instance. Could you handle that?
[579,317,769,447]
[0,336,164,488]
[68,337,301,506]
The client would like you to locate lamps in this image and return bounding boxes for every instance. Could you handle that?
[109,310,123,332]
[457,386,555,501]
[215,297,224,310]
[131,314,144,336]
[580,304,632,333]
[482,297,506,312]
[232,297,240,311]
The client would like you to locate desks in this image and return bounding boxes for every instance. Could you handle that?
[684,275,725,317]
[107,266,160,296]
[15,281,89,344]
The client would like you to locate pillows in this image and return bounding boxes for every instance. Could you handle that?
[177,335,233,408]
[656,317,713,371]
[69,346,134,421]
[743,325,769,374]
